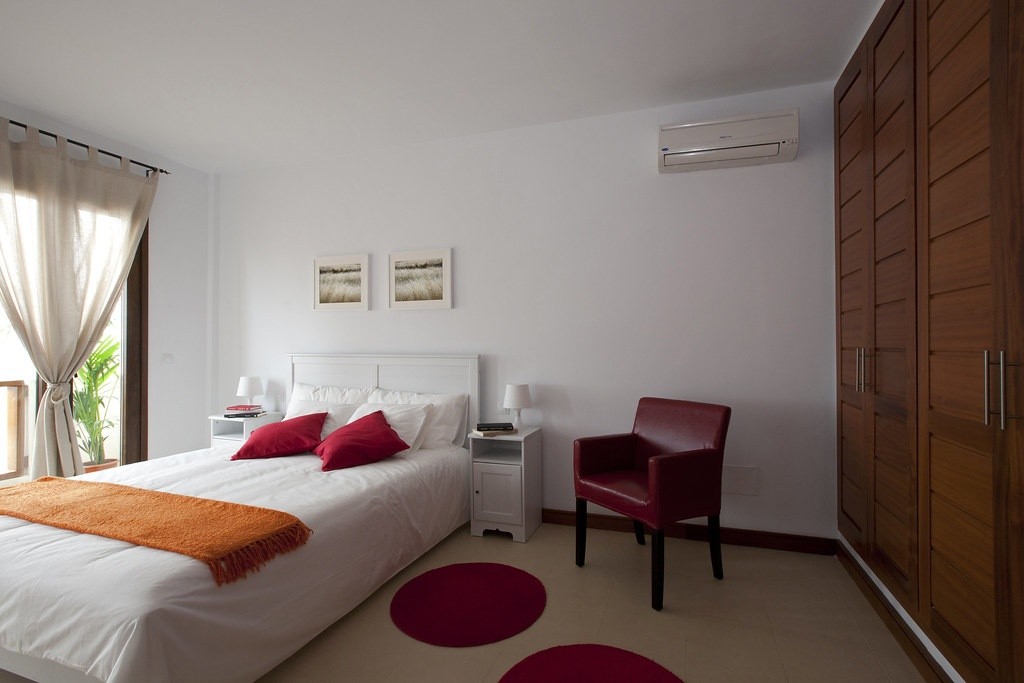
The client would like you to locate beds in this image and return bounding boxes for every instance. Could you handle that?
[0,353,480,683]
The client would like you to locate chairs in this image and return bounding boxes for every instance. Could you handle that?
[574,397,732,612]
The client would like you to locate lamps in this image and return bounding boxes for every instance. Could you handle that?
[504,384,533,429]
[236,377,264,405]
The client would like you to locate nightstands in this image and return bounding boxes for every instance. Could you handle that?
[208,412,283,447]
[467,427,543,544]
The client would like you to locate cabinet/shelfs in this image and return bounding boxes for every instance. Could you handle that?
[835,0,1024,683]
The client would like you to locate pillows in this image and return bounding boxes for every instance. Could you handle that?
[310,410,411,473]
[290,382,370,408]
[280,399,359,442]
[343,402,433,457]
[230,412,328,460]
[367,386,469,450]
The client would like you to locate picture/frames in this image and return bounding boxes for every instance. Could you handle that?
[387,247,452,311]
[313,253,370,312]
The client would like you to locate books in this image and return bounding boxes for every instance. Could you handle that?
[473,429,518,437]
[224,410,262,415]
[227,405,262,411]
[477,423,513,431]
[224,411,268,418]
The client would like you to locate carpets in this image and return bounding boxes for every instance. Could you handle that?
[390,562,547,647]
[498,643,685,683]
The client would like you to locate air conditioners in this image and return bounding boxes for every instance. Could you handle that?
[658,106,800,174]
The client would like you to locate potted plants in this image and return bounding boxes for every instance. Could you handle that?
[72,337,121,473]
